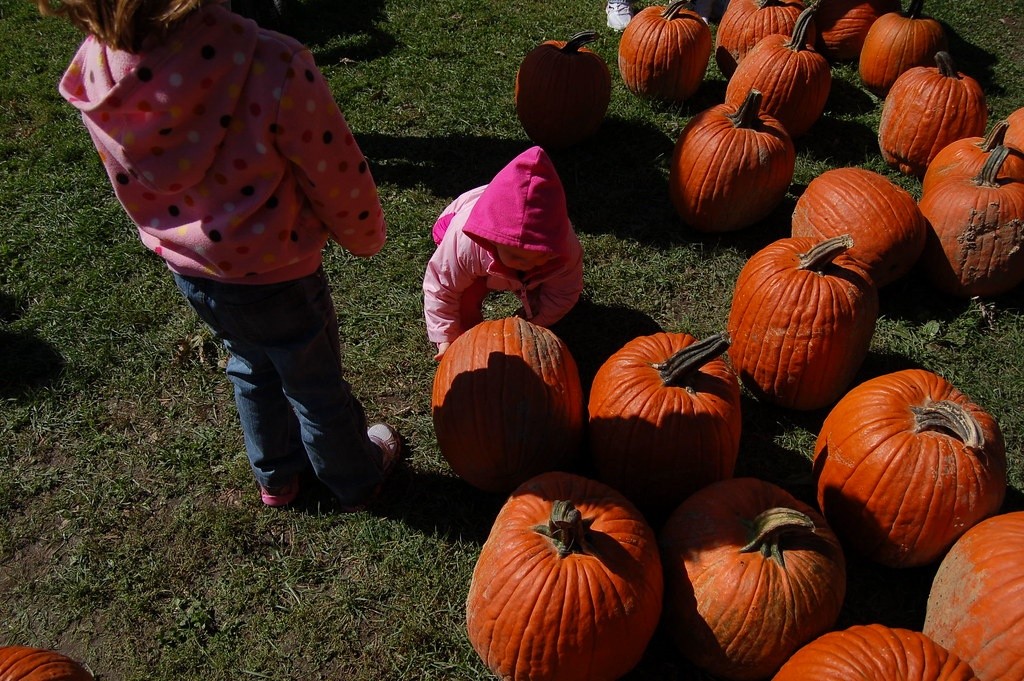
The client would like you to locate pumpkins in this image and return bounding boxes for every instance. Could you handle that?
[0,646,96,681]
[433,0,1024,681]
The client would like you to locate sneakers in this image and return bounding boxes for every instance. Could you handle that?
[605,0,635,33]
[339,422,401,514]
[258,473,301,508]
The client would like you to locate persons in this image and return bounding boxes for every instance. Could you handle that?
[606,0,633,33]
[421,147,582,361]
[40,0,397,506]
